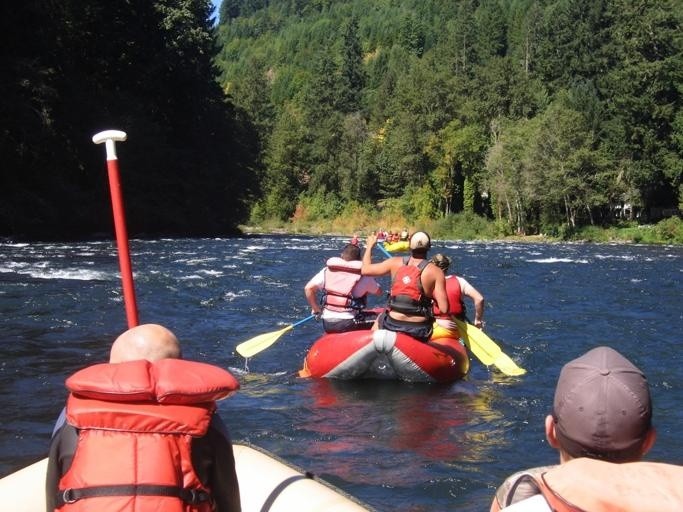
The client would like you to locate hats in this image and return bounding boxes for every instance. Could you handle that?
[553,345,652,452]
[409,232,431,249]
[432,253,449,270]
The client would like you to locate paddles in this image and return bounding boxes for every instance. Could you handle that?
[236,312,316,358]
[495,351,528,376]
[376,242,501,366]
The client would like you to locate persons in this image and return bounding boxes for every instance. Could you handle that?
[484,345,683,512]
[45,323,245,512]
[359,230,449,345]
[427,252,485,331]
[303,244,384,333]
[351,228,410,247]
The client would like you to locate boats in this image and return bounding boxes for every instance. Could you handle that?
[0,427,379,512]
[340,228,413,255]
[293,302,474,386]
